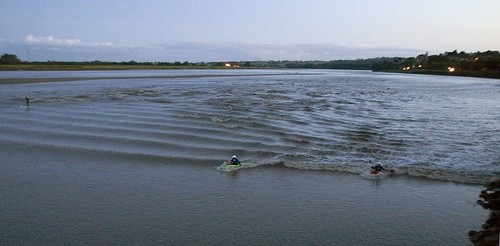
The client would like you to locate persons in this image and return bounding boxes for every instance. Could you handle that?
[230,154,241,165]
[370,162,388,175]
[25,96,29,107]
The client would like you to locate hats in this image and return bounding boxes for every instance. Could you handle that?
[233,155,236,158]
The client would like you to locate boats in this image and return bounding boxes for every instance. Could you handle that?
[220,161,240,170]
[369,170,385,176]
